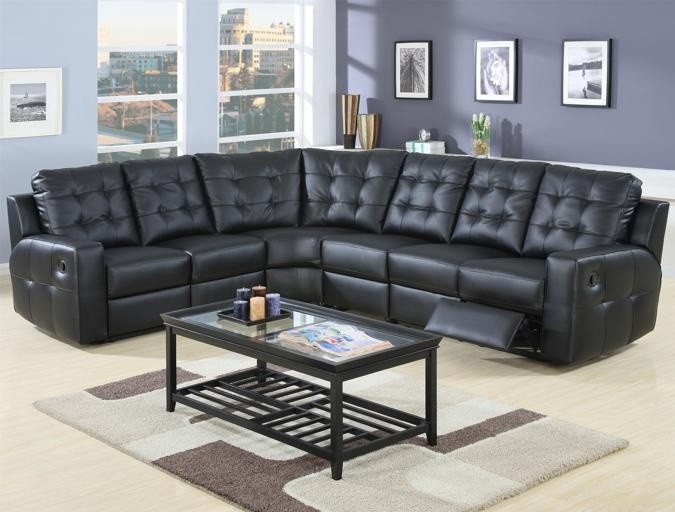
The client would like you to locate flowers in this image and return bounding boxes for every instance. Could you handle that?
[462,112,499,137]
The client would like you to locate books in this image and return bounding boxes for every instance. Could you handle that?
[279,319,393,359]
[403,140,447,156]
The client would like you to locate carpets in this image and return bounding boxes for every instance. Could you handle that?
[30,343,632,512]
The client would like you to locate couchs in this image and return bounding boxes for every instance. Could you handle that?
[5,149,668,365]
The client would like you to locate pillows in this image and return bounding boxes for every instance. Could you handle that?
[450,157,551,258]
[29,161,141,249]
[521,162,643,259]
[301,147,409,235]
[120,153,217,249]
[381,152,476,245]
[191,146,304,233]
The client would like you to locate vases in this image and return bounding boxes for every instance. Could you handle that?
[467,140,492,154]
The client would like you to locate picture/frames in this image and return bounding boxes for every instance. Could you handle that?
[473,35,518,103]
[390,38,432,102]
[558,36,612,109]
[2,65,66,143]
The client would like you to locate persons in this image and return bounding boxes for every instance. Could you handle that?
[579,64,592,97]
